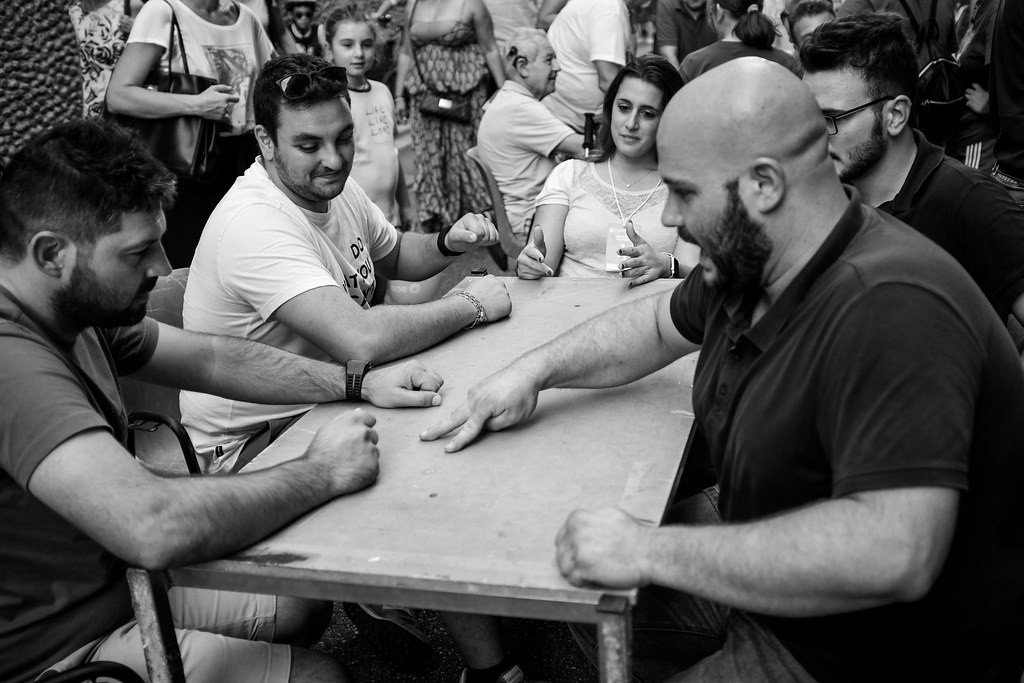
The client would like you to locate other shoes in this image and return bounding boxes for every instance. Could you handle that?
[459,658,532,683]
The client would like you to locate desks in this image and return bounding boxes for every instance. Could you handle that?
[126,277,696,683]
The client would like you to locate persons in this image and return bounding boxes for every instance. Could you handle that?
[1,0,1023,683]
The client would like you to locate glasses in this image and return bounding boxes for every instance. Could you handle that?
[296,12,312,18]
[274,65,349,102]
[823,95,898,135]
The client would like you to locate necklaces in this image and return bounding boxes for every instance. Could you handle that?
[611,160,650,187]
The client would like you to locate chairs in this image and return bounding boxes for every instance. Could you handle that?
[120,268,200,476]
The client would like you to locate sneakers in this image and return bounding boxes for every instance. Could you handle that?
[343,600,432,670]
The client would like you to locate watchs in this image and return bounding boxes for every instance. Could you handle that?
[664,252,680,279]
[345,359,372,401]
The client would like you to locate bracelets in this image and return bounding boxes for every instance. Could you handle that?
[395,96,404,100]
[454,290,487,329]
[437,224,467,257]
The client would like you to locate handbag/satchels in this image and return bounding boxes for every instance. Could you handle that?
[95,1,218,180]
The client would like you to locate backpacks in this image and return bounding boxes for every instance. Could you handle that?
[419,90,472,123]
[898,0,998,152]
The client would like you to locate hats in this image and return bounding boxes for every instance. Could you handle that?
[285,0,316,12]
